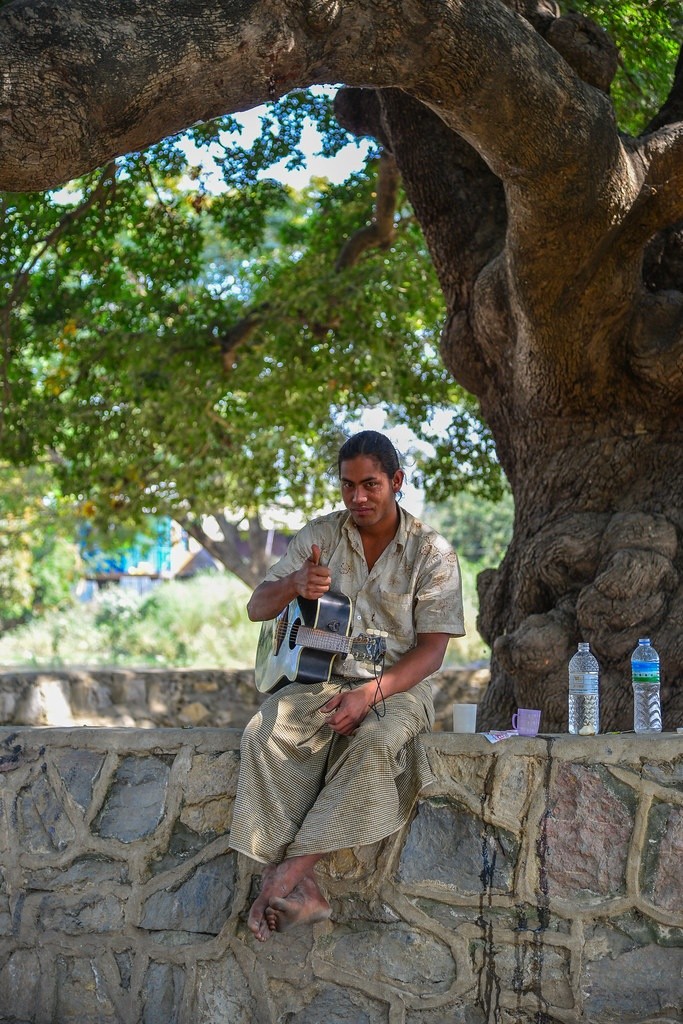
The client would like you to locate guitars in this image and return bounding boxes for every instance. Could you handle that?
[253,590,389,697]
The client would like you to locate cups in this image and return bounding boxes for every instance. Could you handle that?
[512,708,541,738]
[452,703,477,734]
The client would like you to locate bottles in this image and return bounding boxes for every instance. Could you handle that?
[631,638,663,734]
[567,642,600,735]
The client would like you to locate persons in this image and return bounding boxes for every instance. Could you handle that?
[228,430,466,942]
